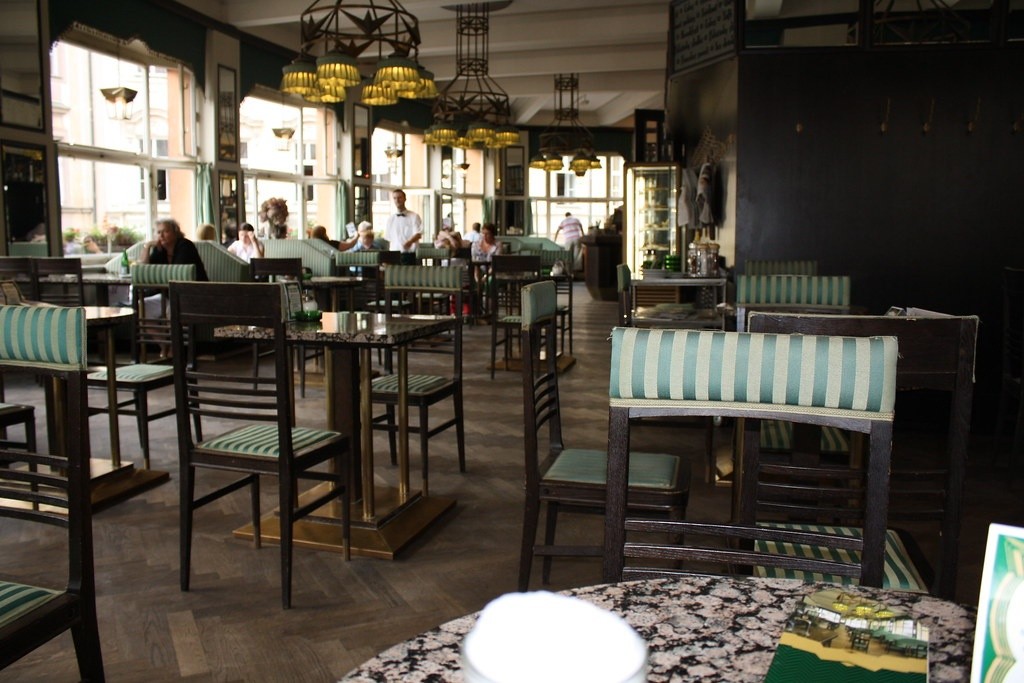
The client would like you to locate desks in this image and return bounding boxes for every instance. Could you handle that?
[487,273,576,375]
[13,272,132,360]
[213,311,457,560]
[335,577,978,683]
[24,305,170,518]
[303,276,365,314]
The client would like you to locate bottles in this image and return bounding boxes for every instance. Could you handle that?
[688,243,719,275]
[302,296,317,313]
[121,248,129,274]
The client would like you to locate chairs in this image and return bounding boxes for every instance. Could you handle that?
[0,251,1024,683]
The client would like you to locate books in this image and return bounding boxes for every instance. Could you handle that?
[762,600,929,683]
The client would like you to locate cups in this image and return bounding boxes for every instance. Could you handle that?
[456,635,649,683]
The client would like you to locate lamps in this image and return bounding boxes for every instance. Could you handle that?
[385,116,403,172]
[99,39,137,121]
[422,2,521,149]
[457,146,469,179]
[279,0,441,106]
[274,94,295,151]
[529,73,602,176]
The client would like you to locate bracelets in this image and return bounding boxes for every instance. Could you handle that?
[144,245,149,248]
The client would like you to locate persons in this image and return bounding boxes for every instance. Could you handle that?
[433,222,504,322]
[385,189,423,266]
[137,218,209,358]
[554,212,584,271]
[228,223,267,281]
[312,221,384,277]
[63,234,104,255]
[197,223,216,241]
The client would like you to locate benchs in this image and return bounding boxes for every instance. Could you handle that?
[105,235,573,361]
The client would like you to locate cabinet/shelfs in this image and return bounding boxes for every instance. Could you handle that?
[623,161,681,272]
[631,273,727,331]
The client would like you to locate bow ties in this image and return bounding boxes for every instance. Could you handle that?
[396,213,405,216]
